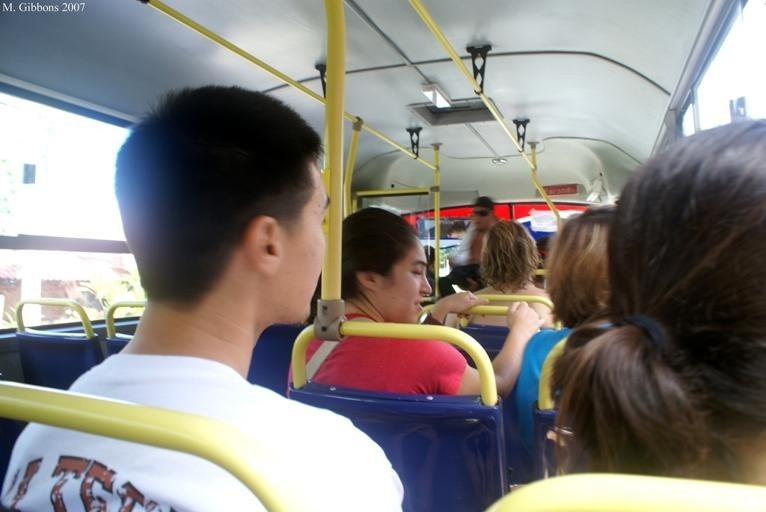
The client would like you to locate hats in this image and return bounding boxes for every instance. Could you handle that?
[472,197,493,208]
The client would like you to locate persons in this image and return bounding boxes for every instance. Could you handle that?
[420,245,476,301]
[517,203,615,463]
[453,218,562,328]
[447,197,502,269]
[553,118,765,487]
[0,82,406,510]
[286,207,545,409]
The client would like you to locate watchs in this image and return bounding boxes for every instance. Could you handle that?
[420,312,442,326]
